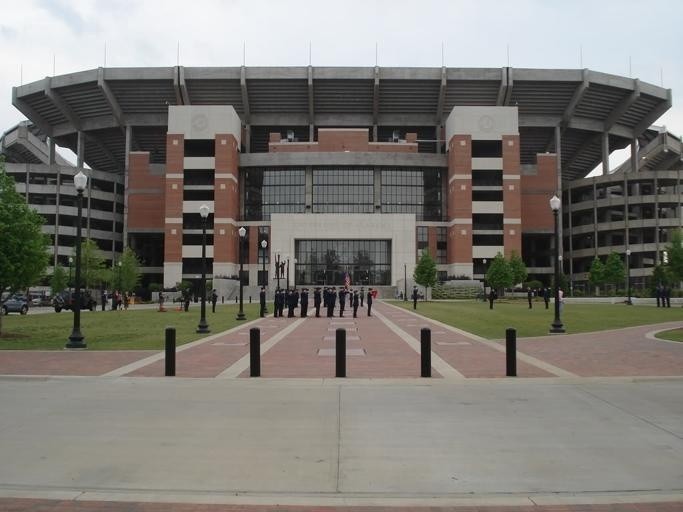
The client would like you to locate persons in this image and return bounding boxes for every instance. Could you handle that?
[558,286,563,312]
[274,260,280,277]
[413,285,418,309]
[279,259,286,278]
[212,289,217,313]
[159,291,164,311]
[656,284,671,306]
[259,286,374,318]
[527,287,532,309]
[99,287,134,311]
[489,289,495,309]
[543,288,549,309]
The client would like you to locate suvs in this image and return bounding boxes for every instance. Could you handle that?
[52,287,97,313]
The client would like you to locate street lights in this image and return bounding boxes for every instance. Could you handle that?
[68,257,74,292]
[625,248,633,305]
[234,226,248,320]
[196,203,211,334]
[548,193,566,333]
[276,250,297,290]
[482,258,487,302]
[117,261,124,292]
[558,255,562,279]
[64,170,89,349]
[261,237,268,313]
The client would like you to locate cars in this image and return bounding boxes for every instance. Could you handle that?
[29,293,53,307]
[0,292,29,315]
